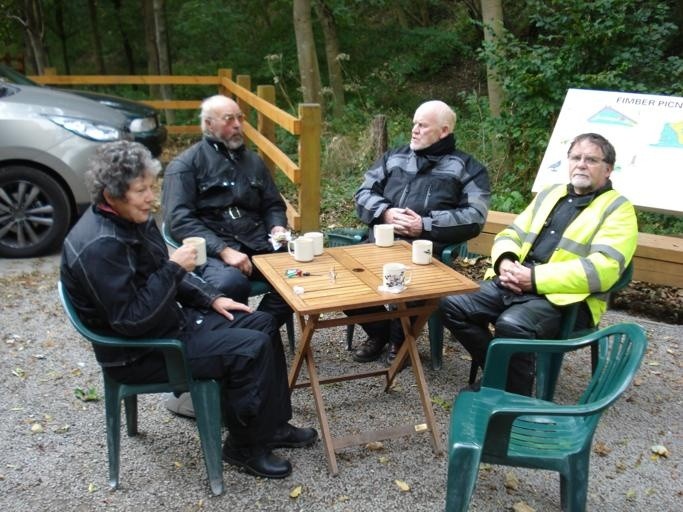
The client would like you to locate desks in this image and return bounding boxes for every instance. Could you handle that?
[251,239,479,477]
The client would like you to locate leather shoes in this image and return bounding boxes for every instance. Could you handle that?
[384,340,406,372]
[220,435,290,478]
[351,336,385,361]
[267,420,317,448]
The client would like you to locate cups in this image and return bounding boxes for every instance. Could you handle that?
[286,236,314,263]
[372,223,394,247]
[304,232,323,256]
[411,239,433,265]
[182,235,207,266]
[382,263,412,291]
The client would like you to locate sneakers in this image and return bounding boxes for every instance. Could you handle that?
[163,389,195,418]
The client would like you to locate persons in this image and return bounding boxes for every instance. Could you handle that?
[339,99,490,368]
[436,132,638,401]
[59,138,318,480]
[162,94,295,328]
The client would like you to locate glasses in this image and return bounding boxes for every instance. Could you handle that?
[210,114,244,123]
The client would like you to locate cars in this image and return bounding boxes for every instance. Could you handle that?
[0,75,135,258]
[0,65,167,159]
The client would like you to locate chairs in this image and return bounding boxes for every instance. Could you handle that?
[56,281,224,496]
[159,218,298,351]
[446,321,648,512]
[345,241,470,372]
[469,256,634,401]
